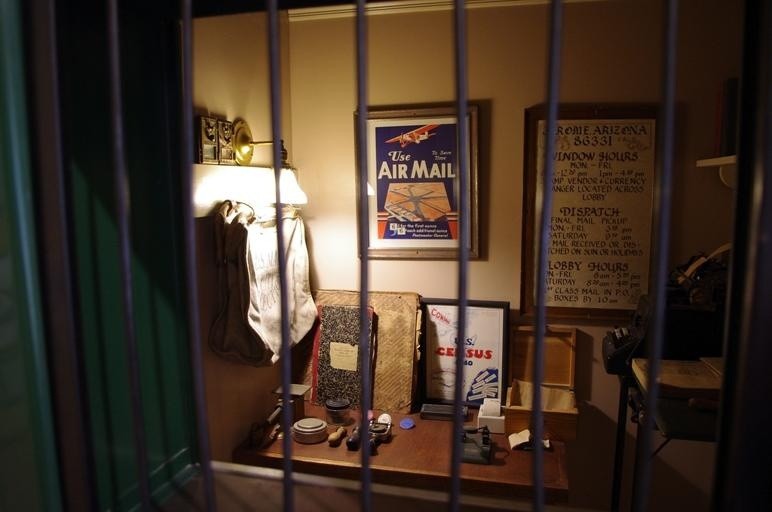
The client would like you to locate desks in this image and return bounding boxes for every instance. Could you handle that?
[610,353,721,502]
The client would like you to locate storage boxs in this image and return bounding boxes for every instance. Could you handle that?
[504,324,578,439]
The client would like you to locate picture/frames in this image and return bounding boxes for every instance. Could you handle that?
[421,298,510,409]
[520,101,664,328]
[355,102,480,261]
